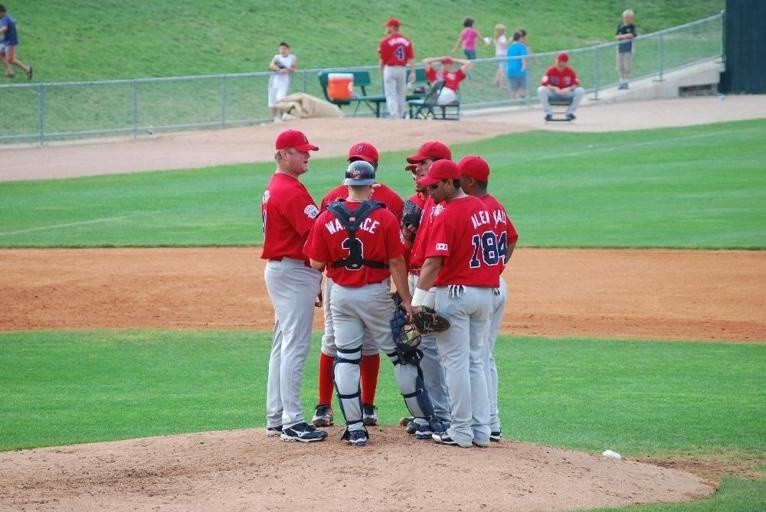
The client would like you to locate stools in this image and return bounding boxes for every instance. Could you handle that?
[545,95,576,122]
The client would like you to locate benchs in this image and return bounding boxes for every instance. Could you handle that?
[315,69,386,118]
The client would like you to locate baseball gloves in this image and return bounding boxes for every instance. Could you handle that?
[412,307,450,333]
[402,199,421,226]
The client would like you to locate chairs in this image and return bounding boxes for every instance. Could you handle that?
[430,66,461,122]
[406,79,447,121]
[404,67,429,101]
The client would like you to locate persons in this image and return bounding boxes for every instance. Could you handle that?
[505,31,529,100]
[517,28,545,69]
[451,16,489,81]
[614,10,640,90]
[491,24,515,90]
[267,41,296,123]
[538,52,585,121]
[260,129,518,446]
[0,3,32,82]
[420,57,474,106]
[376,18,416,120]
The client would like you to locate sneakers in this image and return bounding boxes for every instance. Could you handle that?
[400,417,501,447]
[266,404,377,446]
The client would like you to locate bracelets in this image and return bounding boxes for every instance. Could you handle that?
[410,287,428,307]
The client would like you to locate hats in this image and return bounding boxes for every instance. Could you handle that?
[441,58,451,64]
[405,141,489,185]
[347,143,378,162]
[384,19,399,26]
[558,54,567,61]
[276,129,318,152]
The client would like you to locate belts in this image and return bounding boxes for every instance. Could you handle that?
[270,258,311,266]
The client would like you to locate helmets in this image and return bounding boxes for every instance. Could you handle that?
[342,160,375,186]
